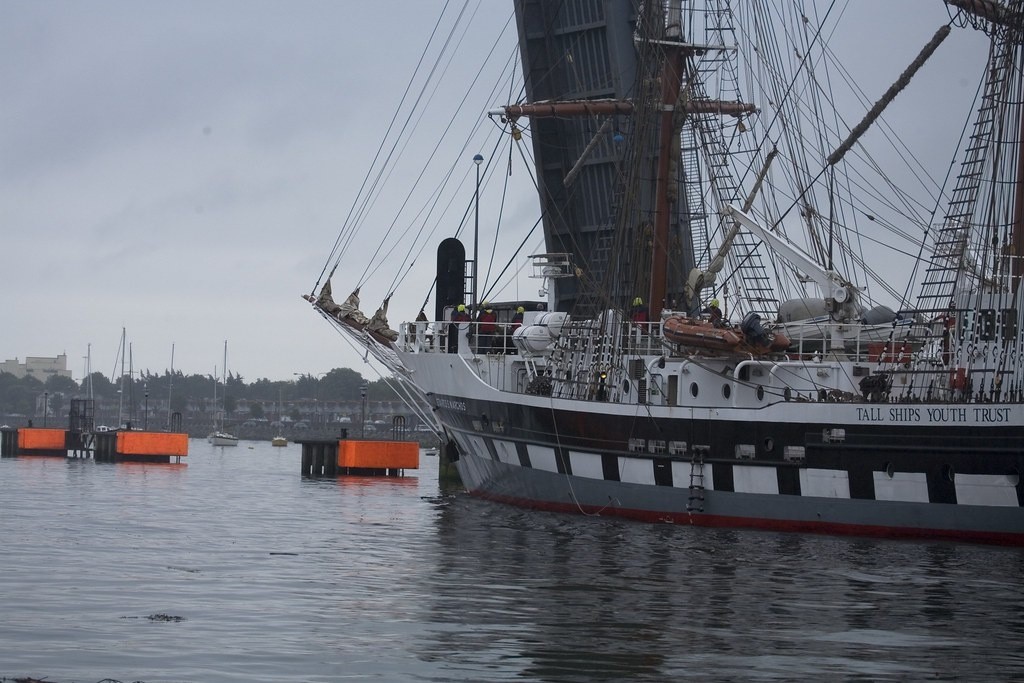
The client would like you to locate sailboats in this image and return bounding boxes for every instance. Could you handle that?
[79,340,240,446]
[272,388,287,448]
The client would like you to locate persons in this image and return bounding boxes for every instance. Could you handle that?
[630,297,648,335]
[451,300,524,355]
[701,298,722,326]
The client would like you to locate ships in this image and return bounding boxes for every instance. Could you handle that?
[304,0,1024,541]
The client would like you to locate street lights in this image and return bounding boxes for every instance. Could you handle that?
[143,386,150,432]
[43,388,49,428]
[472,154,484,321]
[358,387,368,438]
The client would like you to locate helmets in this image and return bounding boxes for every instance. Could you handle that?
[517,306,525,315]
[457,305,466,313]
[482,300,492,308]
[710,299,720,307]
[632,298,643,307]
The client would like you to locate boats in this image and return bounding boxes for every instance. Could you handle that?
[662,317,790,355]
